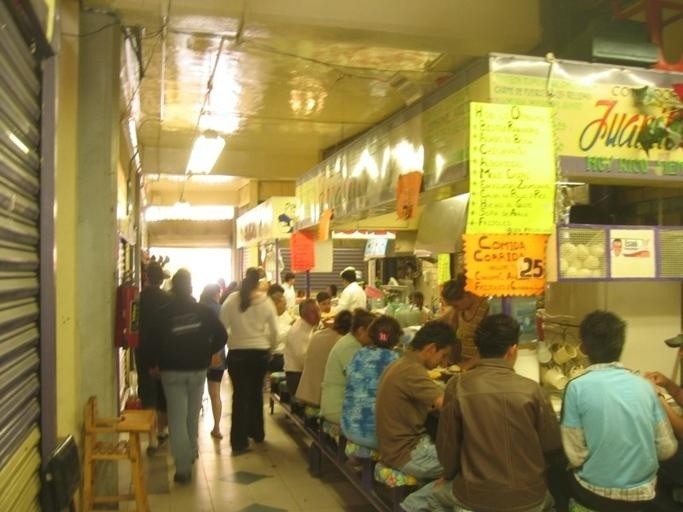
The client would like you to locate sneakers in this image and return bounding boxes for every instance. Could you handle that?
[147,433,169,457]
[174,472,191,484]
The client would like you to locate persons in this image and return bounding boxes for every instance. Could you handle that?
[134,261,492,484]
[398,314,568,512]
[550,308,678,511]
[644,334,683,510]
[611,239,623,256]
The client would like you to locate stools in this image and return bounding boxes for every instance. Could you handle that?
[291,398,304,414]
[306,407,323,431]
[270,372,285,392]
[374,462,423,511]
[279,380,288,401]
[324,421,342,446]
[346,440,380,489]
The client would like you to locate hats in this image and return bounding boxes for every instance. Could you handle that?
[664,334,683,348]
[144,262,170,279]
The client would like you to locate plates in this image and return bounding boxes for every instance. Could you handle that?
[662,393,676,404]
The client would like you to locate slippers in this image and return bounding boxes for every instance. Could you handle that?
[211,431,222,439]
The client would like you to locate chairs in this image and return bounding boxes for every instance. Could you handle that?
[82,395,155,512]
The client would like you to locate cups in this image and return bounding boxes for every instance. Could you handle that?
[552,342,576,365]
[537,340,552,364]
[570,365,584,378]
[545,366,569,390]
[550,392,563,423]
[574,342,589,362]
